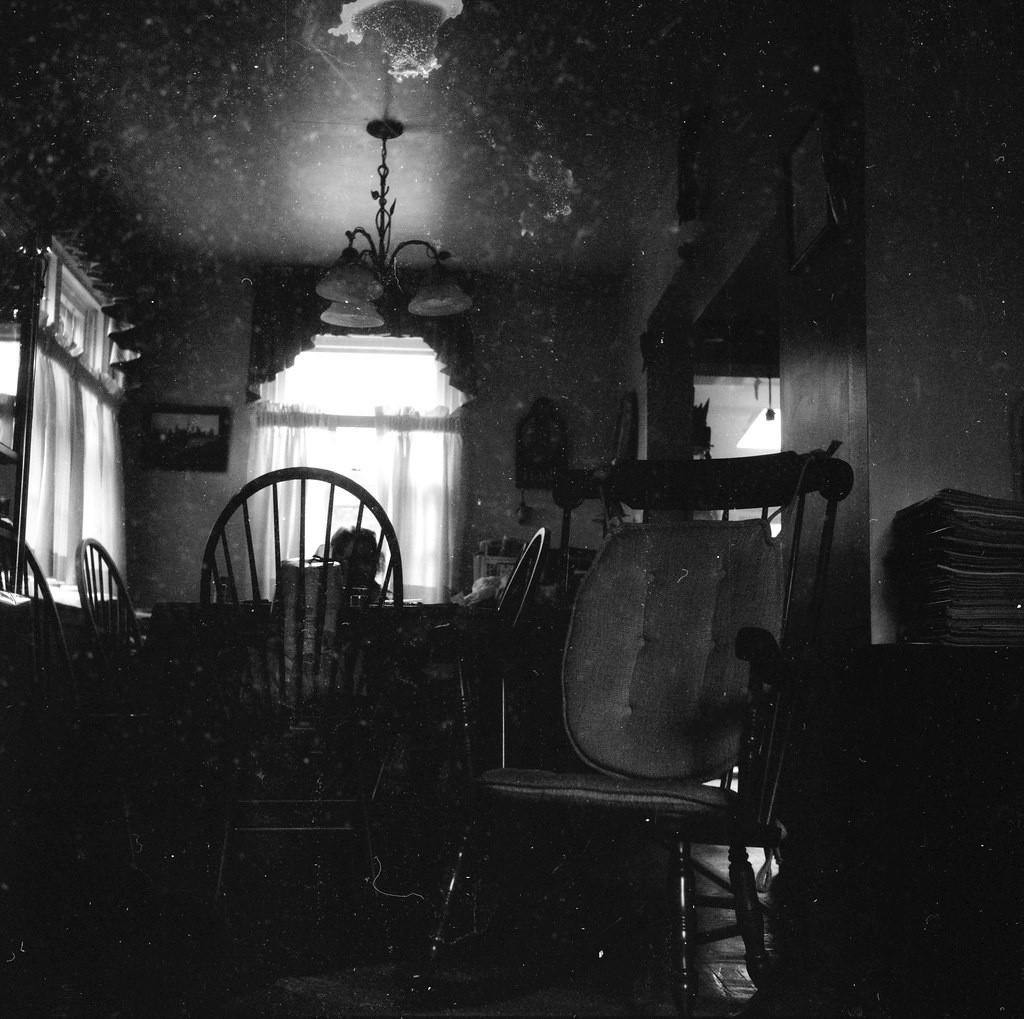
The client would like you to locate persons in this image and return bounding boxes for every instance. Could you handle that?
[334,529,380,602]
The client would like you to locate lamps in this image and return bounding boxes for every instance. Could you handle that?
[313,121,472,327]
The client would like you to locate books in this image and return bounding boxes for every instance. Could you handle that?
[896,488,1024,650]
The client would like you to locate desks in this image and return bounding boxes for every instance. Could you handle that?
[140,602,497,972]
[806,644,1024,1019]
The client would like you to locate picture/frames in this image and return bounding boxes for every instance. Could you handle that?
[140,404,230,471]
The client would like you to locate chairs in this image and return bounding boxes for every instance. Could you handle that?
[21,452,854,1019]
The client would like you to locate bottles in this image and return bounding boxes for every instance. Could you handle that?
[352,586,370,607]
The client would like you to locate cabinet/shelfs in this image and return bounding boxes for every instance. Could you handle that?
[0,252,89,657]
